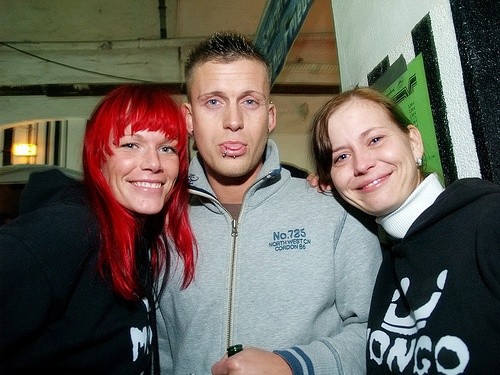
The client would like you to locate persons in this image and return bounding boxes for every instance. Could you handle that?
[148,30,383,375]
[1,81,198,375]
[305,88,500,375]
